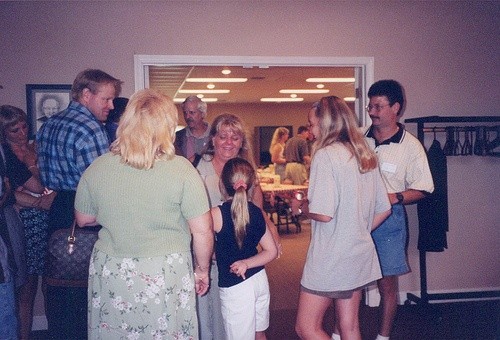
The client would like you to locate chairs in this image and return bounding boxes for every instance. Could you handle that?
[273,195,301,232]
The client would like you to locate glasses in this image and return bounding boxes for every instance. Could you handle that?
[365,103,393,112]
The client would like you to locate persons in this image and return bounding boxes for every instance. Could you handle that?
[270,124,315,185]
[290,95,393,340]
[332,79,435,340]
[0,67,281,340]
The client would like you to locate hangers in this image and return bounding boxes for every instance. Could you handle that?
[433,125,500,157]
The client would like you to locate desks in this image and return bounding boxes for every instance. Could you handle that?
[261,183,308,226]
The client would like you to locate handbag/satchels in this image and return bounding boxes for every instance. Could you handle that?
[43,219,99,287]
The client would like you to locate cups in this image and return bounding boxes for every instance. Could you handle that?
[274,175,280,187]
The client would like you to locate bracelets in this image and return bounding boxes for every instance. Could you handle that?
[28,165,36,170]
[395,192,404,204]
[193,261,211,269]
[298,203,304,216]
[193,273,209,280]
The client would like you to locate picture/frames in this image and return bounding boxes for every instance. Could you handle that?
[26,84,72,140]
